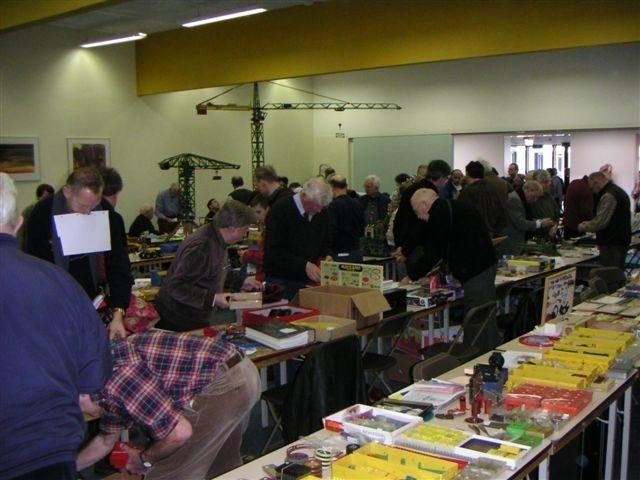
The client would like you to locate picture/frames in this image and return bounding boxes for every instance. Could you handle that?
[0,135,40,182]
[66,138,111,177]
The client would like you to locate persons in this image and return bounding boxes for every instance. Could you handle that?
[18,183,54,251]
[264,176,333,299]
[253,165,293,204]
[507,163,525,198]
[359,174,392,229]
[75,330,262,480]
[330,174,361,233]
[578,172,632,271]
[247,190,269,223]
[525,167,563,224]
[445,169,464,201]
[456,160,491,205]
[504,180,553,248]
[479,157,510,199]
[562,175,594,241]
[22,167,131,339]
[392,188,502,349]
[227,175,253,202]
[205,198,220,224]
[158,200,263,329]
[0,171,110,480]
[129,203,160,239]
[155,183,182,234]
[391,159,451,281]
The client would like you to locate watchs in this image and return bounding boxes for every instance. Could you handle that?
[112,308,125,316]
[138,451,153,468]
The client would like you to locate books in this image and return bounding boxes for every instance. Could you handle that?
[246,316,316,354]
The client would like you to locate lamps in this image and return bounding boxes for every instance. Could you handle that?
[183,7,267,28]
[80,33,148,48]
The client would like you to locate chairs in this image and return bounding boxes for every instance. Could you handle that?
[358,312,408,396]
[419,302,497,359]
[260,334,359,455]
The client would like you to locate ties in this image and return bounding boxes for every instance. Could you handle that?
[304,212,309,221]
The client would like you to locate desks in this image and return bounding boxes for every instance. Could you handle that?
[185,238,640,366]
[215,266,640,480]
[129,246,183,265]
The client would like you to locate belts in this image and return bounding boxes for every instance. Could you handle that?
[225,348,245,369]
